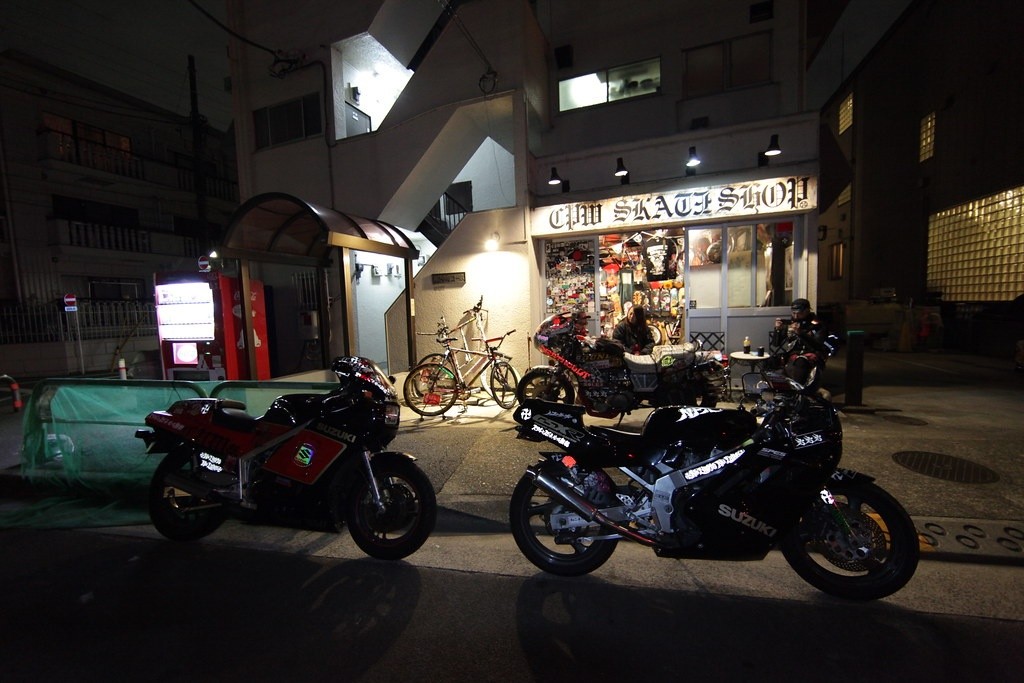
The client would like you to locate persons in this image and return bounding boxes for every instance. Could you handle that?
[760,298,841,396]
[612,305,655,355]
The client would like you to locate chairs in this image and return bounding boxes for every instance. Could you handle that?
[688,332,733,400]
[768,330,786,358]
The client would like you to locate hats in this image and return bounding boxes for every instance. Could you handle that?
[791,299,810,312]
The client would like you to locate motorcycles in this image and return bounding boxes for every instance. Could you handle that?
[134,355,437,560]
[508,368,921,602]
[513,309,731,410]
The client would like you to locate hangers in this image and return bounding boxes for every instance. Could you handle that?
[653,232,663,238]
[624,264,631,270]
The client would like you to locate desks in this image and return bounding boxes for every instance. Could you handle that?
[730,352,770,393]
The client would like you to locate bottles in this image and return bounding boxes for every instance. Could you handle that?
[743,336,751,354]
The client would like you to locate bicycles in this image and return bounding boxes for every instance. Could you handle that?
[401,293,523,421]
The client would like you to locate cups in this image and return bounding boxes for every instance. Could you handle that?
[757,347,765,357]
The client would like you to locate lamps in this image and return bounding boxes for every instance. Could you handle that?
[757,134,782,166]
[684,145,701,176]
[614,157,630,184]
[548,168,569,194]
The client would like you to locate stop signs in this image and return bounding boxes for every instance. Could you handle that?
[64,293,76,306]
[198,255,209,270]
